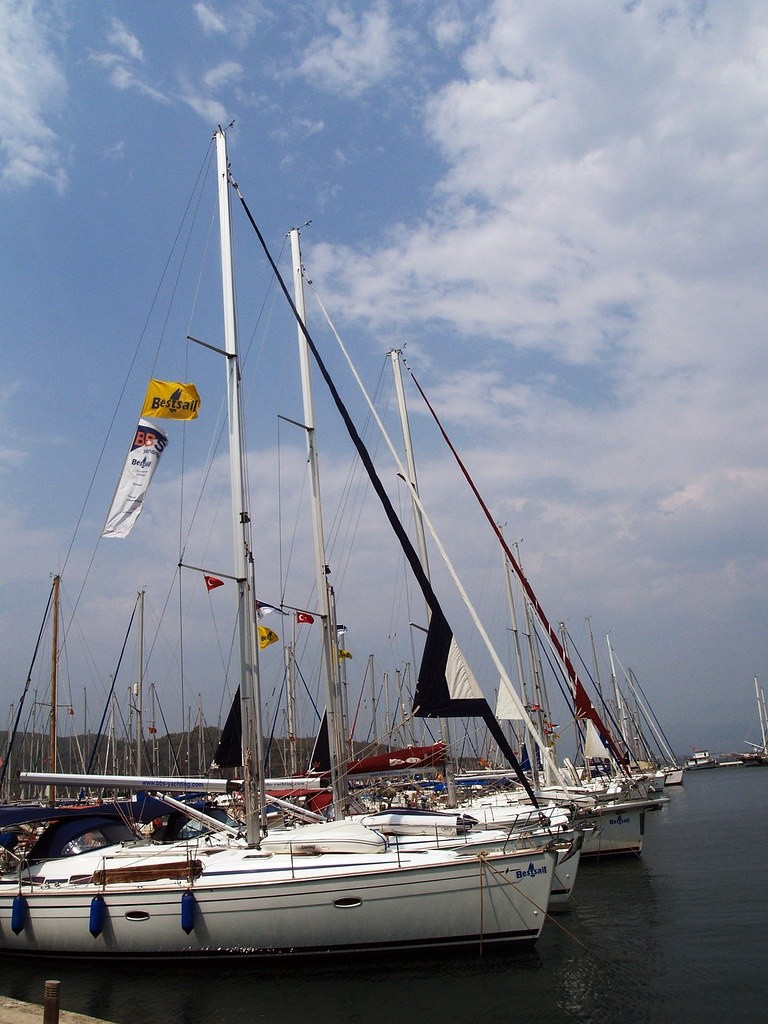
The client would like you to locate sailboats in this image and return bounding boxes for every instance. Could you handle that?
[719,677,768,766]
[0,126,684,962]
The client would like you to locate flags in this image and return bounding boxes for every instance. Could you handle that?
[527,702,560,742]
[258,626,279,651]
[297,612,314,624]
[142,379,201,421]
[103,418,169,539]
[338,649,352,664]
[204,575,224,591]
[255,600,290,621]
[331,625,348,643]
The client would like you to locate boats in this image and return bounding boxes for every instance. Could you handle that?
[683,744,720,770]
